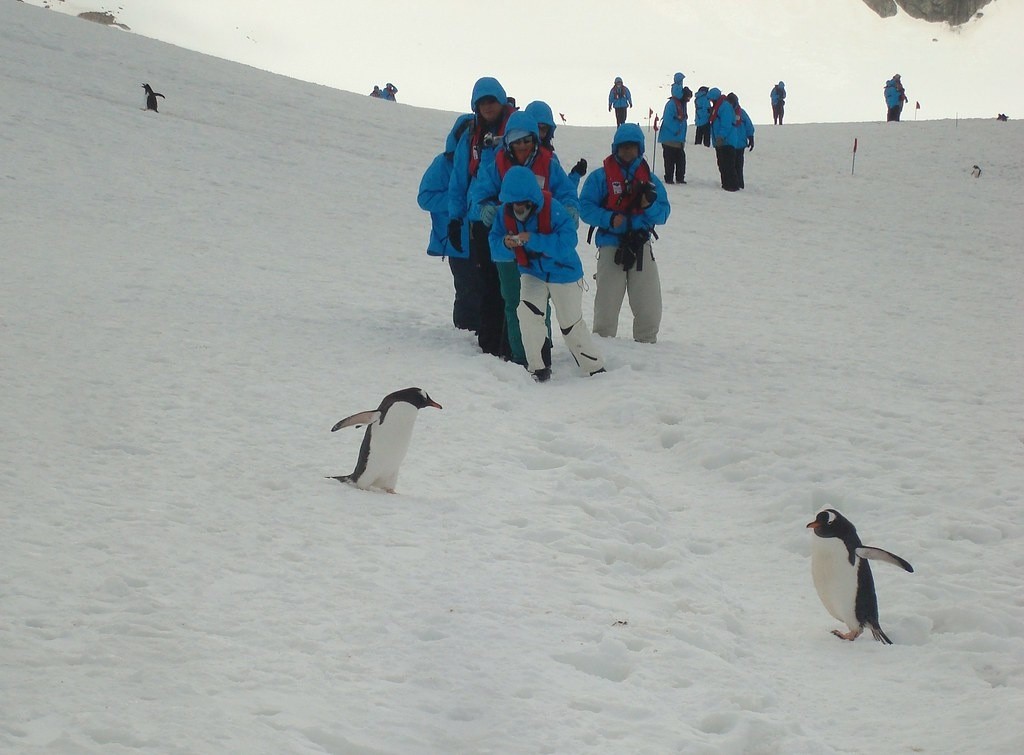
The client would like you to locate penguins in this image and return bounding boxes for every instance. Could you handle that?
[804,507,914,645]
[139,82,166,114]
[970,164,982,179]
[322,387,443,497]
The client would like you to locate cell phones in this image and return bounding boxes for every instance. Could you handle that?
[510,235,525,246]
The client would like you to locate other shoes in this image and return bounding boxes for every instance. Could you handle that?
[532,368,552,382]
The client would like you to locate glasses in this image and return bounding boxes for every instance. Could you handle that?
[512,136,533,144]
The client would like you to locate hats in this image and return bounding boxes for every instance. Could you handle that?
[698,87,709,93]
[684,87,693,98]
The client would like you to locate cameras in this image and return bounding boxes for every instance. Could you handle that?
[483,133,502,147]
[634,181,658,208]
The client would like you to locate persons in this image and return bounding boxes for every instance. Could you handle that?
[578,123,671,344]
[609,77,632,129]
[418,78,606,382]
[370,83,398,102]
[884,74,908,121]
[657,72,755,192]
[770,81,786,125]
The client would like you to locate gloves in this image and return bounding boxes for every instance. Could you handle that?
[716,137,723,147]
[518,232,529,243]
[504,235,518,248]
[571,158,587,176]
[480,205,501,227]
[447,219,465,255]
[748,136,754,151]
